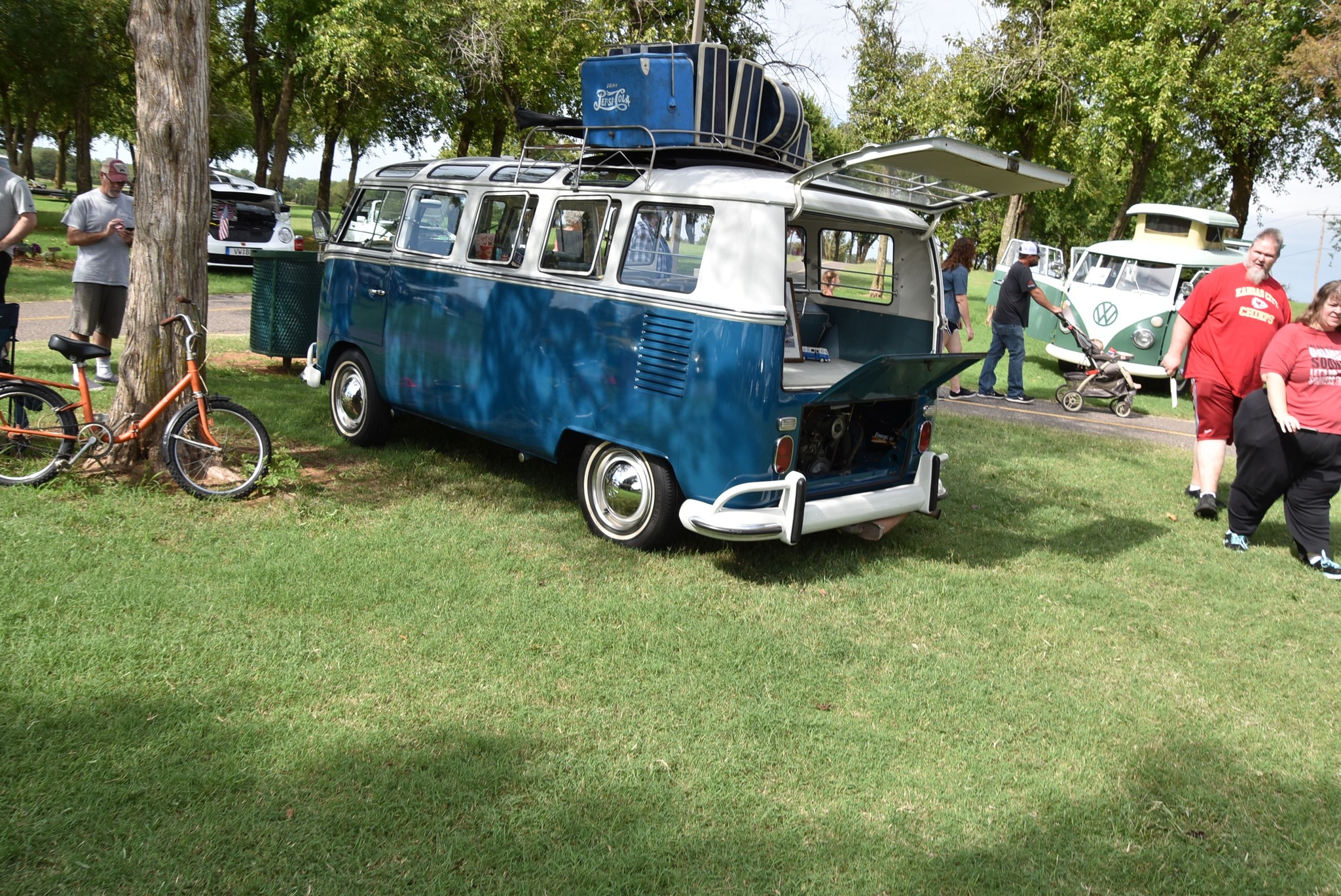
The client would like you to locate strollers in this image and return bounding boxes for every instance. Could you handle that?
[1055,313,1136,418]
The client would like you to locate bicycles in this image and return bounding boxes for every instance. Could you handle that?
[0,295,272,502]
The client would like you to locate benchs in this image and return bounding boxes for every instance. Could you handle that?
[559,260,594,269]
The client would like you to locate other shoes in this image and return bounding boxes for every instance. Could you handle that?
[977,390,1005,399]
[948,386,976,400]
[95,374,120,385]
[71,375,105,392]
[937,396,944,400]
[1195,494,1217,520]
[1005,394,1035,403]
[1184,486,1227,507]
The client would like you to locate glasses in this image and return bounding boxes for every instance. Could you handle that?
[103,174,126,185]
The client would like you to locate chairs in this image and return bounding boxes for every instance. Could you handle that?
[502,246,582,260]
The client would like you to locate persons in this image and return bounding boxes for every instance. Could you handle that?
[552,210,585,254]
[787,227,791,239]
[61,158,136,391]
[1161,228,1292,520]
[822,270,840,296]
[1090,339,1141,389]
[522,214,530,244]
[622,207,672,278]
[0,166,37,362]
[130,176,135,197]
[1223,280,1341,579]
[938,237,975,401]
[977,242,1064,403]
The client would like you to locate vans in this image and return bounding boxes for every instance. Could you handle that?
[986,204,1286,397]
[314,137,1072,546]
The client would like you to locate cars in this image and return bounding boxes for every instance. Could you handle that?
[207,168,296,268]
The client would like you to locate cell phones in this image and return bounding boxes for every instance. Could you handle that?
[124,227,135,233]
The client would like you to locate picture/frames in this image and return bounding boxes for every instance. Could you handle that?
[783,276,804,363]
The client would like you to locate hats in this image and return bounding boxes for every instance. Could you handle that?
[1019,241,1046,257]
[101,157,134,188]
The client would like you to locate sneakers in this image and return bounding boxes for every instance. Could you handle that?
[1302,549,1341,580]
[1223,529,1248,553]
